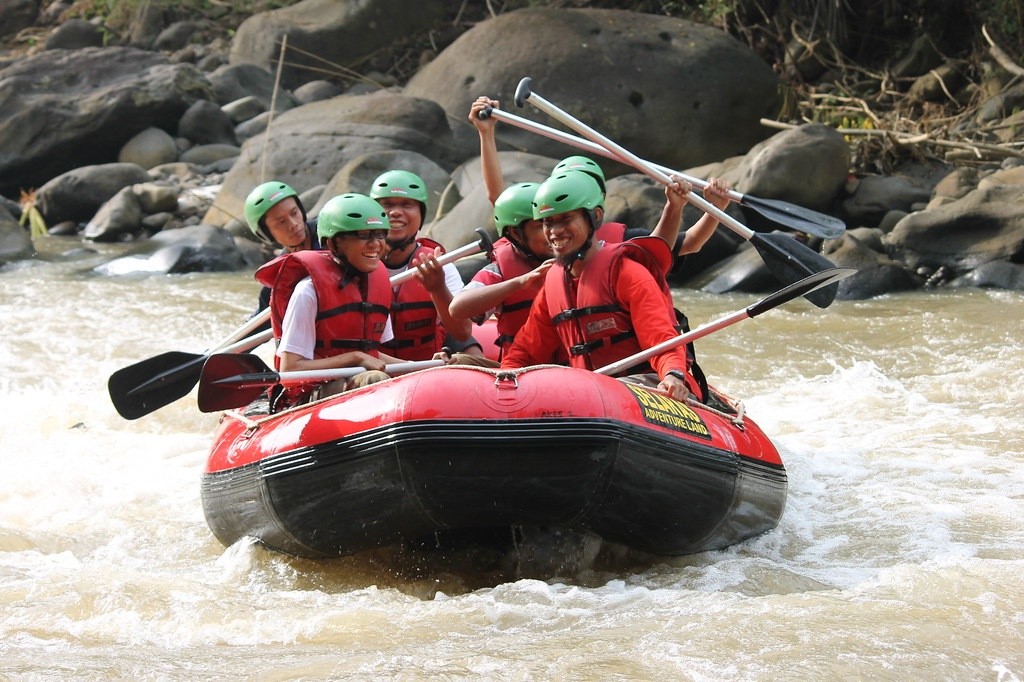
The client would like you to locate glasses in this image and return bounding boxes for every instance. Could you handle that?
[340,229,389,239]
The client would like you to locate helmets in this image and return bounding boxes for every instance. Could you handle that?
[244,179,307,247]
[552,155,607,198]
[317,192,391,250]
[532,172,605,231]
[492,182,545,237]
[369,170,428,232]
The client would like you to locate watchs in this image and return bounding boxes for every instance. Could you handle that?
[666,370,685,384]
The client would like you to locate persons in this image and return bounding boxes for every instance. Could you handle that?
[498,170,702,402]
[447,171,691,370]
[232,179,319,413]
[469,95,731,257]
[368,169,472,378]
[278,192,396,398]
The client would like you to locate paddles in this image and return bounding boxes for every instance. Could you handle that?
[202,306,273,356]
[512,76,842,309]
[197,346,454,414]
[108,227,495,421]
[477,101,847,240]
[592,266,860,377]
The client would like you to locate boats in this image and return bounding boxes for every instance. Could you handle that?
[201,318,788,559]
